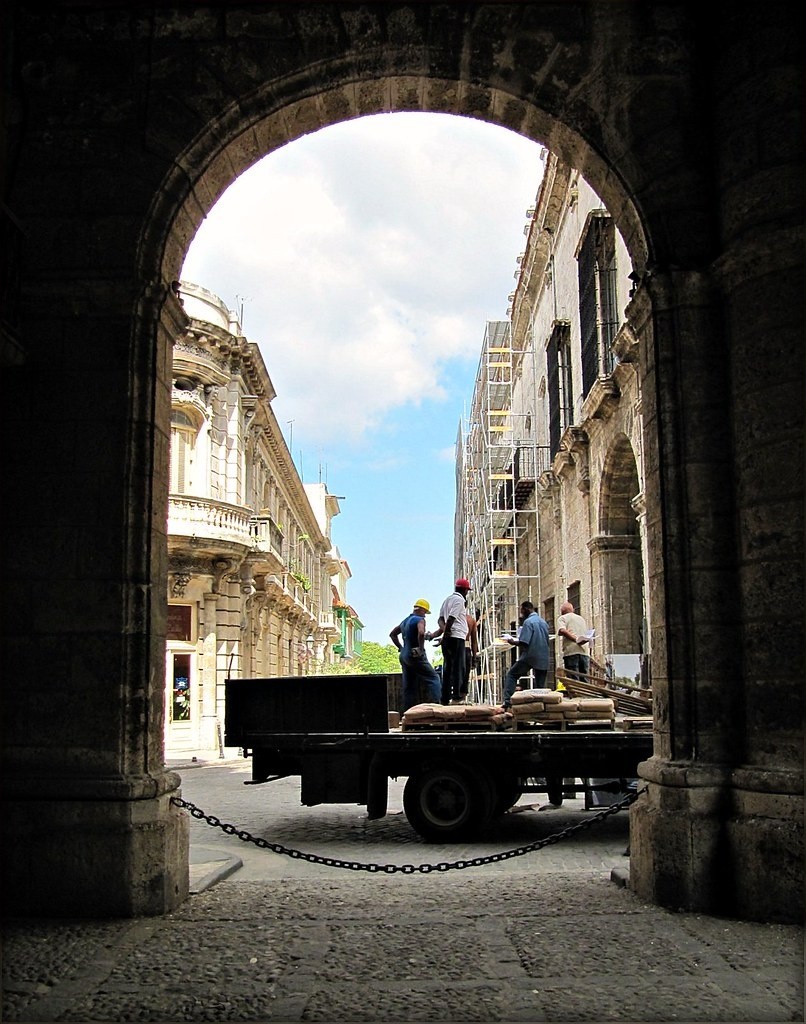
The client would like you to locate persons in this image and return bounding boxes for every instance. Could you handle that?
[390,579,478,719]
[556,602,591,696]
[501,601,551,708]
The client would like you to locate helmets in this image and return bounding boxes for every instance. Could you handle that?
[414,599,431,614]
[455,579,472,590]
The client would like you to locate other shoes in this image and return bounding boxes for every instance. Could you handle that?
[451,699,472,705]
[501,703,512,712]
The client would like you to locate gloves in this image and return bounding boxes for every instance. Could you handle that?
[412,646,425,657]
